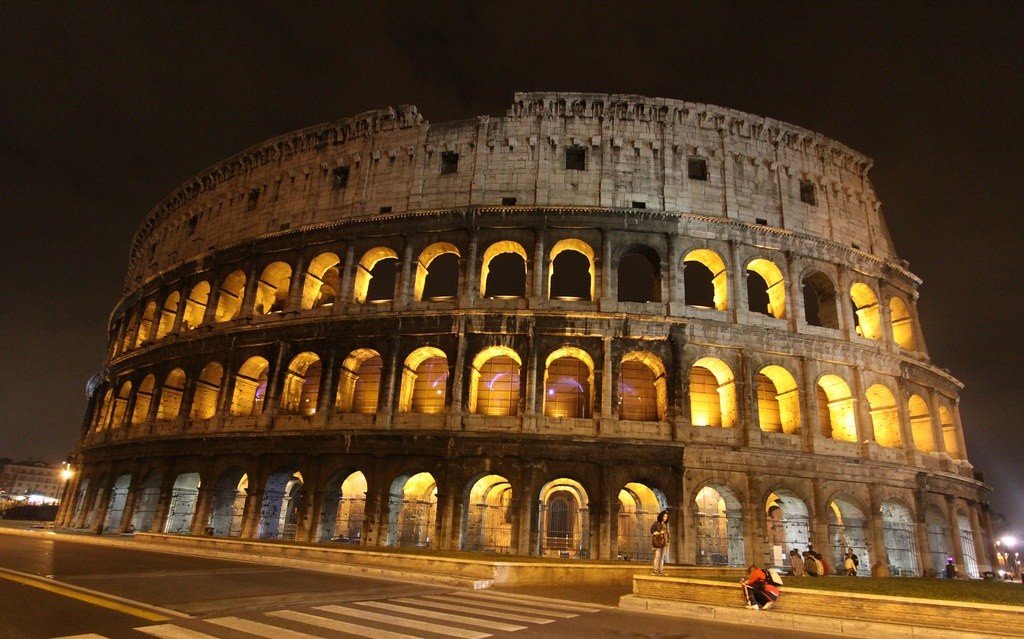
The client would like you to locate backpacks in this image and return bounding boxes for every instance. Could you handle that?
[761,568,784,586]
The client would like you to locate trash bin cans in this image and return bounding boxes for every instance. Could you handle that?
[983,571,995,580]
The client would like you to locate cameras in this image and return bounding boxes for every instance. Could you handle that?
[740,578,745,584]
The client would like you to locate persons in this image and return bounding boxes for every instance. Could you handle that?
[787,548,859,577]
[945,560,956,579]
[651,512,671,576]
[742,565,780,610]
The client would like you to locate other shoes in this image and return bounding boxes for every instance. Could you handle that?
[745,605,759,610]
[658,570,668,576]
[762,602,773,610]
[651,570,657,575]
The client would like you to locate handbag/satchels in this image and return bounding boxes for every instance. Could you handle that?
[652,534,665,548]
